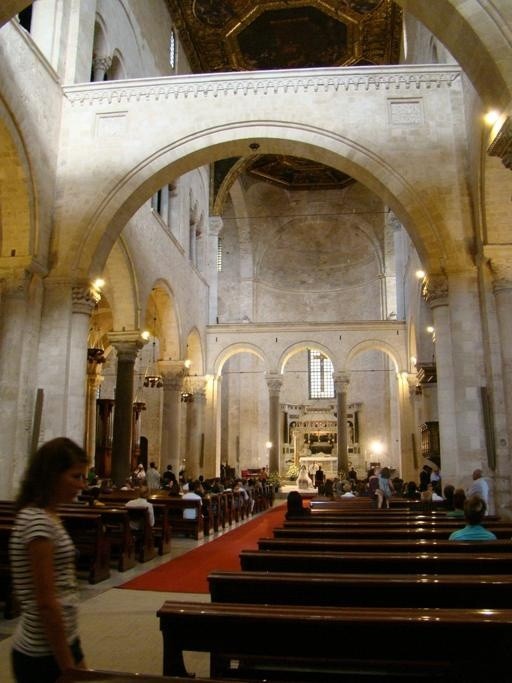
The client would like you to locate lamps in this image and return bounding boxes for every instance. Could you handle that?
[180,344,195,403]
[143,305,163,388]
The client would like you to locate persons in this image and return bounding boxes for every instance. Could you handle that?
[9,438,88,681]
[315,466,466,516]
[285,492,310,519]
[296,465,312,489]
[83,462,271,534]
[465,468,491,516]
[448,495,497,542]
